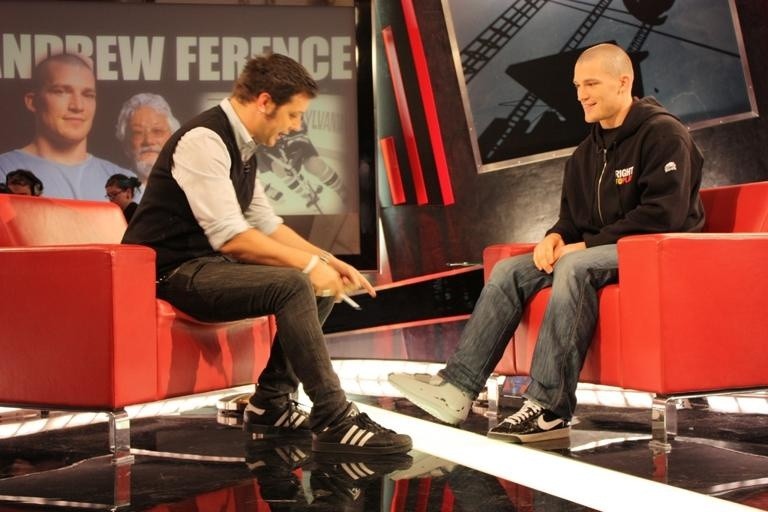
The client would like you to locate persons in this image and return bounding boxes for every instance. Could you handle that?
[105,174,141,226]
[2,53,145,204]
[116,95,183,185]
[249,457,414,512]
[253,119,355,215]
[386,42,706,443]
[390,445,514,511]
[117,55,412,455]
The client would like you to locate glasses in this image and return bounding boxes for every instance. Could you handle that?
[105,190,123,198]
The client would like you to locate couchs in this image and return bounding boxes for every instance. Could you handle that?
[477,180,768,455]
[569,434,767,500]
[0,456,261,512]
[0,192,287,455]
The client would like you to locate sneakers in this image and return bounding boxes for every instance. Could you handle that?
[389,452,457,480]
[487,400,570,444]
[311,403,412,454]
[243,441,313,475]
[310,454,412,500]
[263,185,284,202]
[304,185,323,206]
[243,399,310,438]
[388,372,473,425]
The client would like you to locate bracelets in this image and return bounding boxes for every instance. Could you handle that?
[318,251,334,265]
[300,255,320,276]
